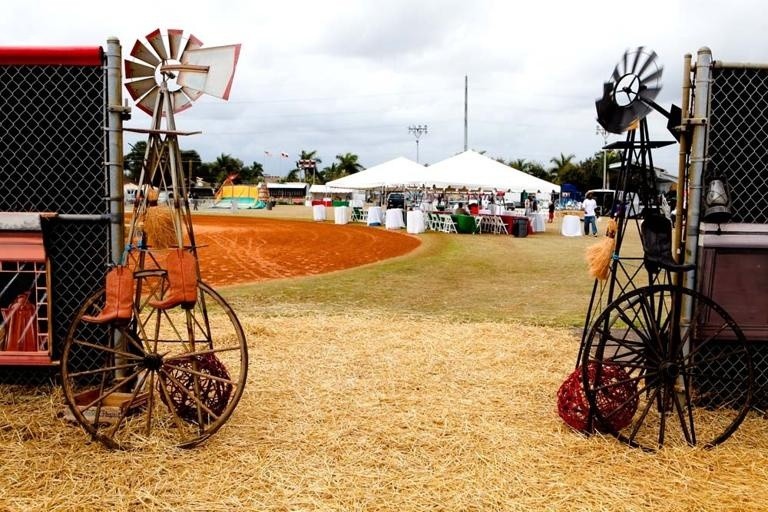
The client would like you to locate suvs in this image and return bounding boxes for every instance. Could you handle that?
[468,191,516,212]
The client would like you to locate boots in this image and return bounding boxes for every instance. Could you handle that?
[641,216,696,273]
[80,265,134,326]
[148,250,197,309]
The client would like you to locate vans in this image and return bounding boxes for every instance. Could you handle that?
[386,192,445,211]
[583,189,640,218]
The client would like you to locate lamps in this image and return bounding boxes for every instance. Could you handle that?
[702,158,731,219]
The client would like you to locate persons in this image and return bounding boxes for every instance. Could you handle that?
[665,183,677,230]
[531,194,541,212]
[582,192,599,238]
[192,191,201,213]
[546,199,556,224]
[660,190,667,206]
[455,202,471,216]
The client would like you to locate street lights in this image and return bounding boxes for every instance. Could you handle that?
[595,125,611,189]
[408,123,428,163]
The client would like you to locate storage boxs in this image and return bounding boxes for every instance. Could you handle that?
[64,388,149,425]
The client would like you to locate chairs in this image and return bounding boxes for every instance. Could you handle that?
[424,205,510,236]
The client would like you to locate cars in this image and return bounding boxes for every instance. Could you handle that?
[157,190,182,205]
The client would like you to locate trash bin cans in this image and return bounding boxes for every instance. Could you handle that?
[267,203,272,210]
[513,217,528,237]
